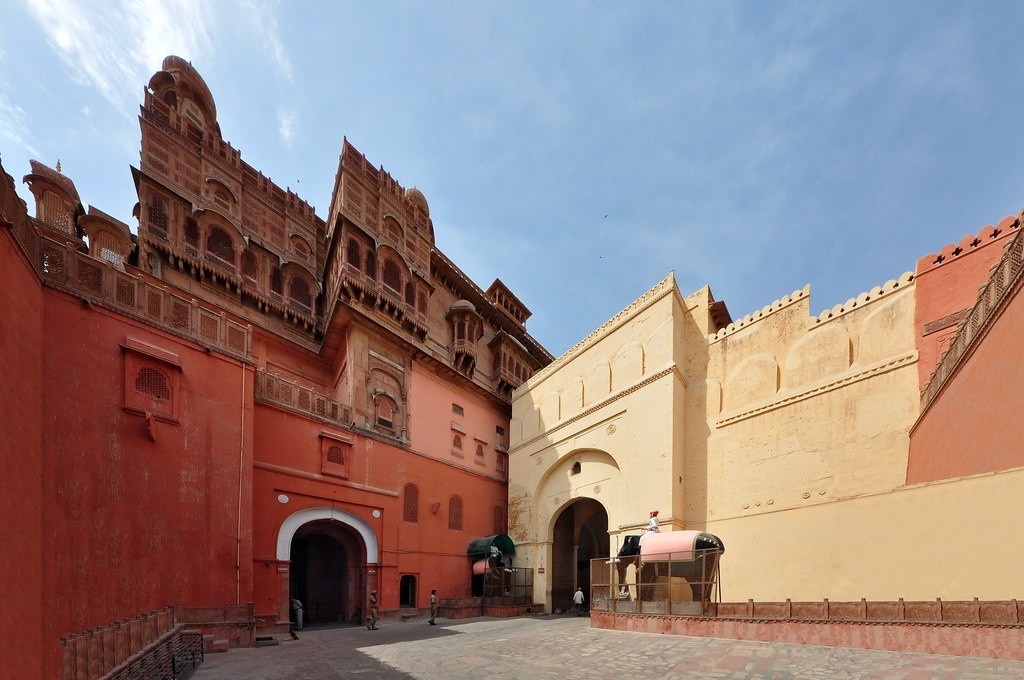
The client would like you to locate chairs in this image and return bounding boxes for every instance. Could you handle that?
[60,604,205,680]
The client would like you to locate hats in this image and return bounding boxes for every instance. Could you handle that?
[371,590,376,593]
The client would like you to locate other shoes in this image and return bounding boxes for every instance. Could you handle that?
[373,628,379,630]
[367,626,371,630]
[432,623,437,625]
[428,620,432,624]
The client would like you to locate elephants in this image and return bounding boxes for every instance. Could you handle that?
[612,531,726,618]
[472,555,518,599]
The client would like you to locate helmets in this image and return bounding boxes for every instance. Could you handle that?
[650,511,659,517]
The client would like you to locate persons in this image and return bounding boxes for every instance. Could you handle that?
[290,597,303,630]
[573,588,584,617]
[639,511,661,546]
[367,590,379,630]
[490,542,500,558]
[428,590,438,625]
